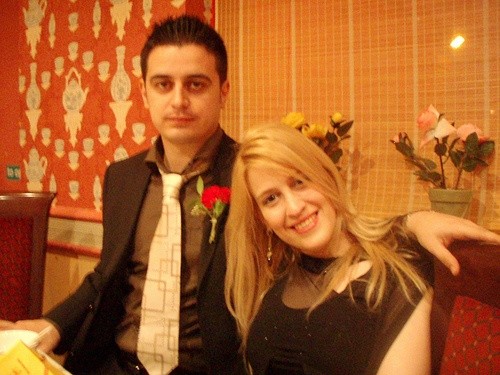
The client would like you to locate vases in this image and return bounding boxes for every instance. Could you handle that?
[428,189,474,219]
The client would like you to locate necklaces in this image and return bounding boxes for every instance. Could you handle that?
[296,262,335,288]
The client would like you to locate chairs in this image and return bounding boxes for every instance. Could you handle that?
[0,191,58,322]
[426,238,500,375]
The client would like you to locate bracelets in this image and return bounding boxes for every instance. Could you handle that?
[401,209,430,239]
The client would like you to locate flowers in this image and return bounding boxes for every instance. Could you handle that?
[389,103,497,189]
[188,176,232,244]
[280,111,354,163]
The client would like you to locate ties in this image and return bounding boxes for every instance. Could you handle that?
[137,158,210,375]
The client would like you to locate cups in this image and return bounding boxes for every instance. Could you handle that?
[0,330,39,352]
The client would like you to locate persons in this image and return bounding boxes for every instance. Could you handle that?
[0,14,500,375]
[224,123,434,375]
